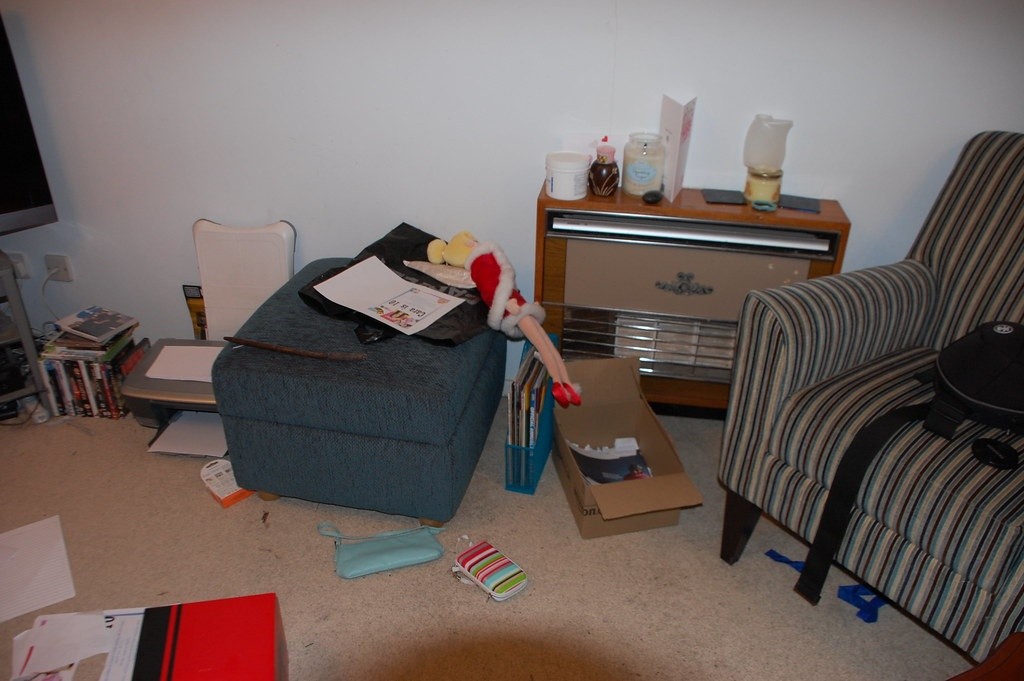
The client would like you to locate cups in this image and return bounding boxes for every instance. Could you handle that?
[621,131,665,199]
[545,151,593,201]
[744,165,783,203]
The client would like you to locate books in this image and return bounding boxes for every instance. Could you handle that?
[507,345,549,490]
[38,305,151,420]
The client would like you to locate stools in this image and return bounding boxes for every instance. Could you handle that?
[210,257,509,529]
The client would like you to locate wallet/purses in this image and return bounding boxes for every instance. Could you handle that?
[454,542,527,601]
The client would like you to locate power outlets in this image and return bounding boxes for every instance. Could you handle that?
[44,253,73,282]
[4,251,33,280]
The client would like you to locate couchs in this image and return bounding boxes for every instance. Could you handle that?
[715,131,1024,666]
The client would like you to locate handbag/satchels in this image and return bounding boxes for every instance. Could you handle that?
[923,321,1024,442]
[297,222,492,347]
[318,524,445,578]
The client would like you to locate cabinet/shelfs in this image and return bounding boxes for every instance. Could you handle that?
[534,178,851,421]
[0,249,52,418]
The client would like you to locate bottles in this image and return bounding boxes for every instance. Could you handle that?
[588,144,620,198]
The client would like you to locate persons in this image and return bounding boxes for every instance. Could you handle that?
[399,316,417,327]
[429,231,581,407]
[391,310,408,321]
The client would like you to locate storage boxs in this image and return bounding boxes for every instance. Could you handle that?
[553,355,705,541]
[201,459,257,509]
[123,338,230,430]
